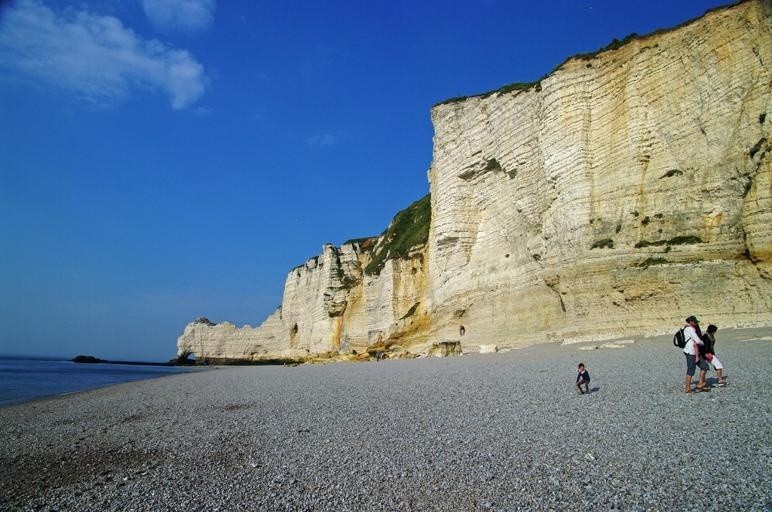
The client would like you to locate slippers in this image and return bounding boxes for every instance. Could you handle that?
[696,381,729,392]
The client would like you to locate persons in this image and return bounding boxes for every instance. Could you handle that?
[576,363,590,394]
[700,325,728,384]
[682,316,710,393]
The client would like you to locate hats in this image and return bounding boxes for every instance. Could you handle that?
[686,316,700,322]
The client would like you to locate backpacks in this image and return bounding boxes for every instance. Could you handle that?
[674,326,690,348]
